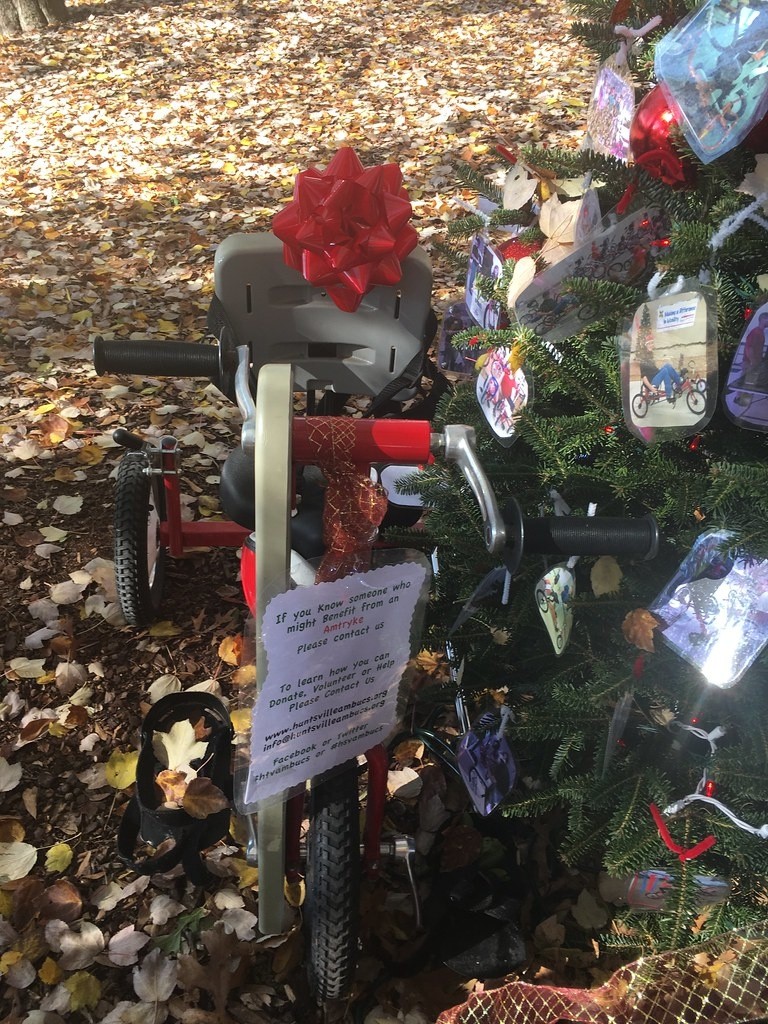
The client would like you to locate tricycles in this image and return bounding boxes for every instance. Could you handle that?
[672,360,707,393]
[90,146,661,1024]
[632,368,706,418]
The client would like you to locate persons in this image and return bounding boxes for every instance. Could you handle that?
[640,336,686,403]
[585,0,663,165]
[742,312,768,376]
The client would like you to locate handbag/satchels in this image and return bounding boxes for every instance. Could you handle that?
[116,691,234,888]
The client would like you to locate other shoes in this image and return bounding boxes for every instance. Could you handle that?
[667,398,676,403]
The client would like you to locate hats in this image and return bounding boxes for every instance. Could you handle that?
[645,335,655,346]
